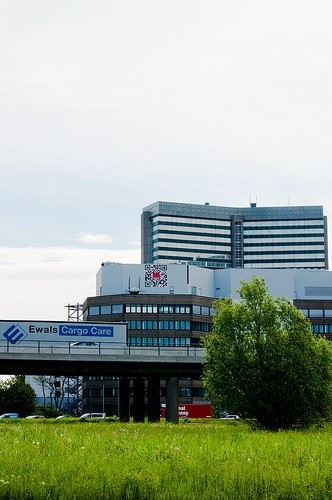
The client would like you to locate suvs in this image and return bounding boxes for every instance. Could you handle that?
[220,415,240,422]
[1,413,18,422]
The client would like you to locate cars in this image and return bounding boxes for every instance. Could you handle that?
[25,414,46,421]
[56,413,72,420]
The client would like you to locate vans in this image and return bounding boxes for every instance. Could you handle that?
[80,412,106,421]
